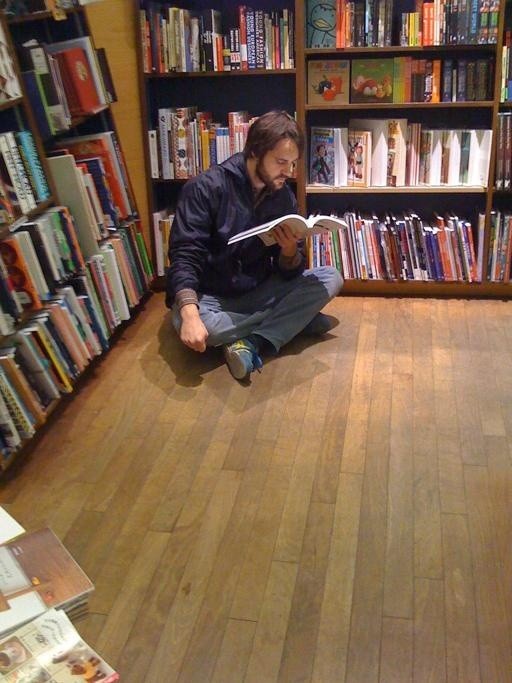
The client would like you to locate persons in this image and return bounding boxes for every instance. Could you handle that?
[163,108,345,382]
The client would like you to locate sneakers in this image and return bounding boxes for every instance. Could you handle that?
[295,314,332,338]
[222,337,263,380]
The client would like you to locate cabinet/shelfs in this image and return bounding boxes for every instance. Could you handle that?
[139,3,512,296]
[2,1,153,486]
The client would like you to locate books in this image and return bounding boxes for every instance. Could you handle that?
[0,496,124,682]
[158,0,349,285]
[306,0,511,103]
[0,1,157,465]
[306,113,512,285]
